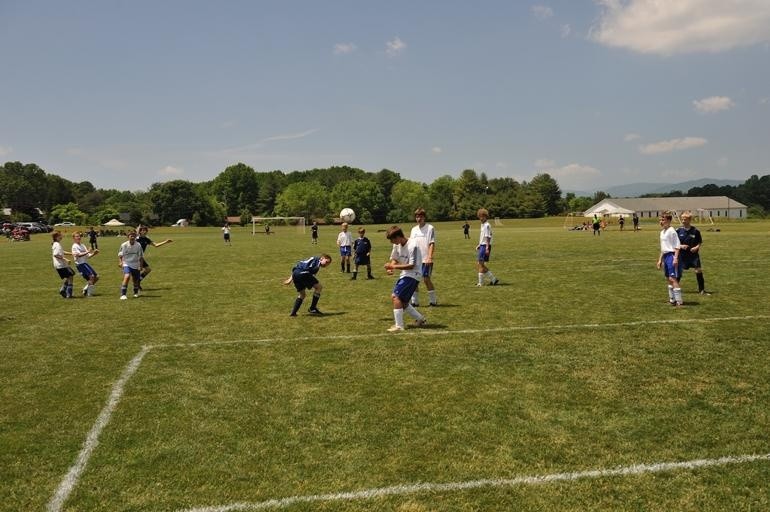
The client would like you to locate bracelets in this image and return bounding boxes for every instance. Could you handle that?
[673,256,678,259]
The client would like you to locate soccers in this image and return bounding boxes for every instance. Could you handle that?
[339,207,356,223]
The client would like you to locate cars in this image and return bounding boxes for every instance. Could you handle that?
[17,221,53,233]
[55,221,76,227]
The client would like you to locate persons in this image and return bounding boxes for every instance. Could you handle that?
[476,209,499,287]
[265,224,269,235]
[284,254,332,316]
[410,209,437,307]
[77,228,136,238]
[350,228,375,280]
[657,212,683,306]
[618,215,625,231]
[71,233,99,297]
[222,223,232,246]
[336,223,355,274]
[85,227,100,251]
[118,230,143,300]
[676,213,712,296]
[1,223,54,241]
[462,222,471,239]
[136,224,172,290]
[51,230,75,299]
[384,226,427,332]
[311,222,318,244]
[633,215,639,232]
[592,214,600,236]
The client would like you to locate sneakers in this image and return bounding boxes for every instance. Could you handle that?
[341,270,345,273]
[138,285,142,290]
[406,319,427,327]
[664,301,676,305]
[367,275,375,279]
[120,295,128,300]
[66,295,77,298]
[468,237,471,239]
[464,238,467,239]
[349,277,357,280]
[699,290,712,295]
[311,242,314,245]
[83,288,87,297]
[411,303,419,307]
[474,282,486,287]
[225,245,227,247]
[489,279,499,285]
[134,293,139,298]
[308,307,322,314]
[427,303,438,307]
[88,292,96,297]
[290,312,300,316]
[60,289,67,298]
[229,245,232,247]
[347,270,350,273]
[386,325,405,333]
[315,242,318,245]
[672,303,685,308]
